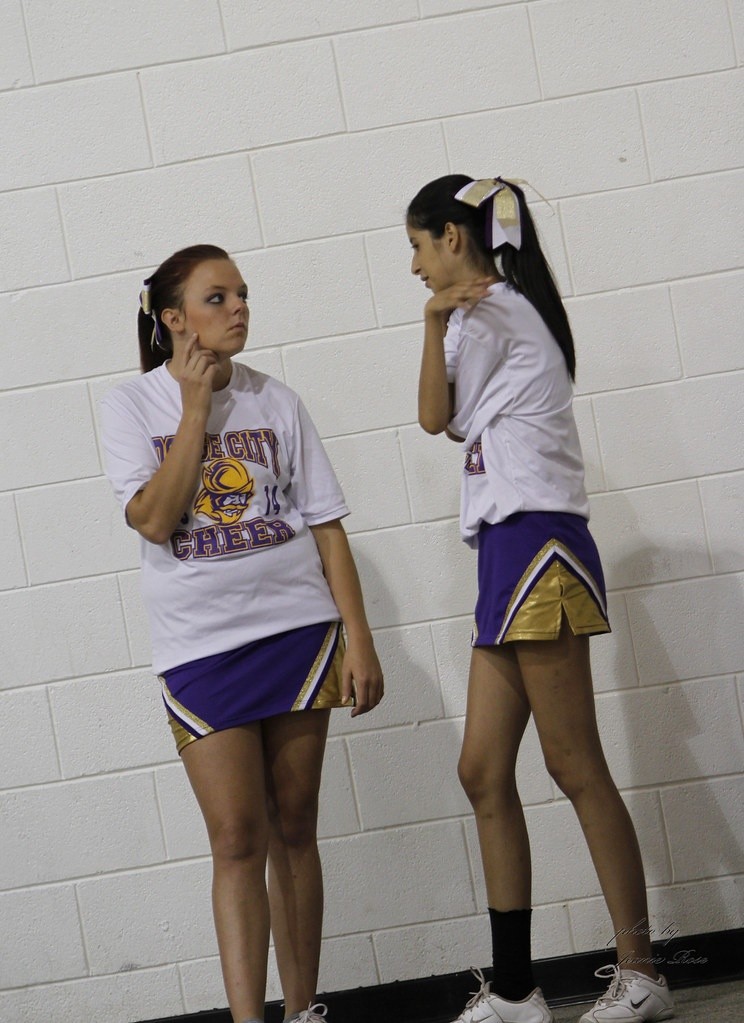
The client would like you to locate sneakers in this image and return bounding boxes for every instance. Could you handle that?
[579,964,675,1023]
[449,966,554,1023]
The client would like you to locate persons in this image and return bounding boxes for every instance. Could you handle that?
[402,174,676,1016]
[103,244,383,1016]
